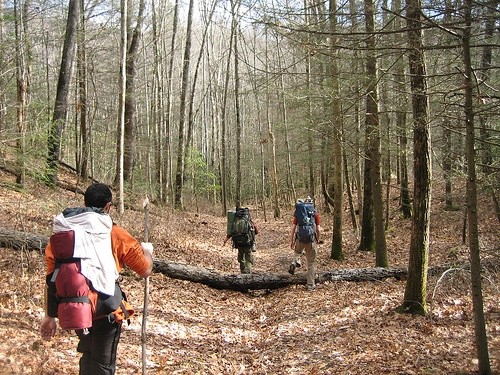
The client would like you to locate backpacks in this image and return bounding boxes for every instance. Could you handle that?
[296,202,316,243]
[45,207,123,334]
[227,207,255,248]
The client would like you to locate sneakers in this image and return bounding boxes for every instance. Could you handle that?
[309,284,316,292]
[288,261,296,275]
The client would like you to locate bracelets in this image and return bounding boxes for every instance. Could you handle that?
[223,241,227,244]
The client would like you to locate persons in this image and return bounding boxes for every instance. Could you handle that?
[40,183,153,375]
[288,198,321,292]
[222,208,260,274]
